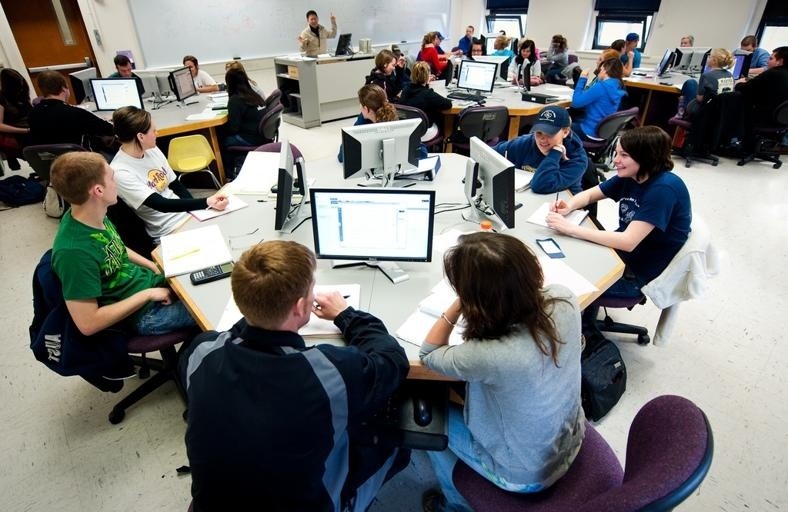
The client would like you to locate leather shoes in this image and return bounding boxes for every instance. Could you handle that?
[412,392,433,425]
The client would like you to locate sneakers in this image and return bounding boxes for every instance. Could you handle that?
[422,488,448,511]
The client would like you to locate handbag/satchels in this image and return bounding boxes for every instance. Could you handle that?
[0,148,47,208]
[43,181,66,219]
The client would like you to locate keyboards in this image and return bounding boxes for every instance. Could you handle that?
[448,91,487,101]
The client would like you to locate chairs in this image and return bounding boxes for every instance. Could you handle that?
[33,248,202,428]
[230,90,286,151]
[579,105,640,185]
[666,90,739,168]
[447,388,715,510]
[580,224,694,350]
[447,104,511,163]
[386,100,429,149]
[724,96,788,170]
[254,141,307,164]
[0,113,33,164]
[21,141,86,184]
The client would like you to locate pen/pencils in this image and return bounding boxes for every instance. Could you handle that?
[505,150,507,159]
[256,200,268,202]
[315,295,350,308]
[556,191,559,214]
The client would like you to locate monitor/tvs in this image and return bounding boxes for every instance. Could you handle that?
[445,55,458,90]
[514,58,531,92]
[470,56,510,87]
[457,60,498,96]
[657,47,754,79]
[461,135,516,234]
[309,187,436,284]
[471,32,518,56]
[342,117,423,188]
[67,66,199,111]
[274,126,312,234]
[335,33,355,55]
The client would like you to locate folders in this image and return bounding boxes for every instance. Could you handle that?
[522,93,560,104]
[394,155,441,182]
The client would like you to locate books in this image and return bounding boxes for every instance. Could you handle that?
[396,277,467,347]
[526,202,590,230]
[159,182,361,340]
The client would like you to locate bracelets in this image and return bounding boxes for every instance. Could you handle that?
[440,313,456,328]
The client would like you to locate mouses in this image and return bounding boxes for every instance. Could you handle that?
[478,100,486,105]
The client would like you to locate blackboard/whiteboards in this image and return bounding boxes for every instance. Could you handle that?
[126,0,453,70]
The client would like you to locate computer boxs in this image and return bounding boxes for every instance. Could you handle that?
[395,379,450,451]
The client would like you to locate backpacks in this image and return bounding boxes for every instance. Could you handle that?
[580,323,626,423]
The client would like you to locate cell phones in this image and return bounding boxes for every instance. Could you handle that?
[660,81,673,86]
[190,260,235,286]
[634,72,646,76]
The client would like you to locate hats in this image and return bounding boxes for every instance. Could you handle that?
[529,104,572,136]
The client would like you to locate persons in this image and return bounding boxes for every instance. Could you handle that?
[174,240,411,512]
[337,25,642,197]
[109,105,229,247]
[669,34,788,121]
[0,51,266,180]
[50,151,199,337]
[545,125,692,299]
[297,10,337,59]
[418,231,587,511]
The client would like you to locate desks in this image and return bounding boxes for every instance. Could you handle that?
[149,152,628,429]
[429,77,574,154]
[68,92,230,186]
[623,71,687,127]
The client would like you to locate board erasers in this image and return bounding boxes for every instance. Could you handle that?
[233,57,241,60]
[401,41,407,43]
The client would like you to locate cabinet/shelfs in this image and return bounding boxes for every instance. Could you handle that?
[273,55,322,128]
[316,53,375,123]
